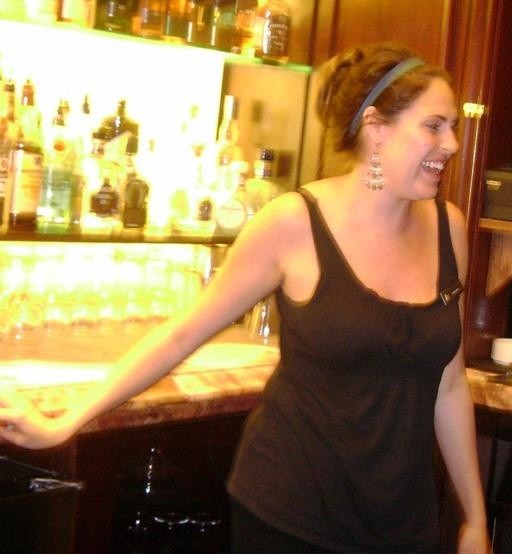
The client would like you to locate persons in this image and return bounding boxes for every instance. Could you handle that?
[0,40,491,554]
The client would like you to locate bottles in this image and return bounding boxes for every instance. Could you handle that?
[0,0,290,231]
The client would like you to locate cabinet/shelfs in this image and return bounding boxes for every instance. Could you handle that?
[4,2,313,436]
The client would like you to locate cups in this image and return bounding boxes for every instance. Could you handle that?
[490,339,512,368]
[245,299,273,344]
